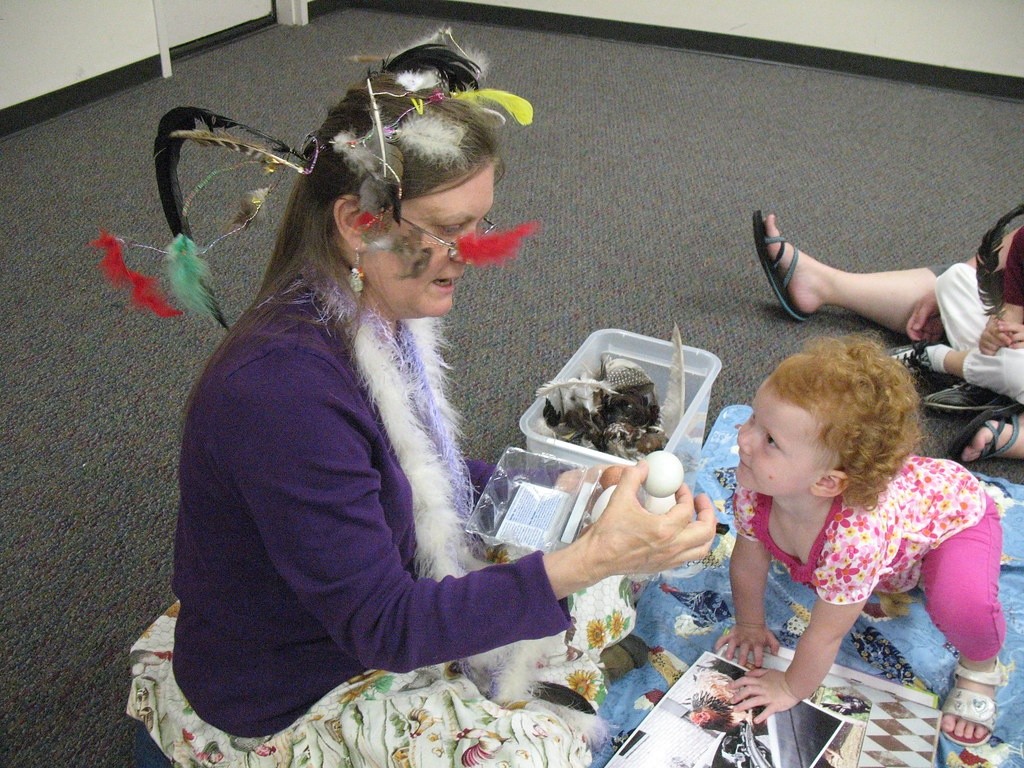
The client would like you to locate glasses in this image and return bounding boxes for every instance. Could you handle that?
[298,130,349,197]
[379,205,498,262]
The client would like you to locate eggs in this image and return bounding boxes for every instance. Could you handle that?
[555,451,684,526]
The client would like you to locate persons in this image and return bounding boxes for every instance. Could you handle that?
[125,36,717,767]
[751,203,1024,464]
[712,331,1010,748]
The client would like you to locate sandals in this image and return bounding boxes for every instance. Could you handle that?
[940,656,1005,747]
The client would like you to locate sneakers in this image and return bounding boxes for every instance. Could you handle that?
[888,339,933,381]
[923,381,1017,411]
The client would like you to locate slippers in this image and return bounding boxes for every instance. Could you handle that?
[753,210,819,320]
[948,402,1024,463]
